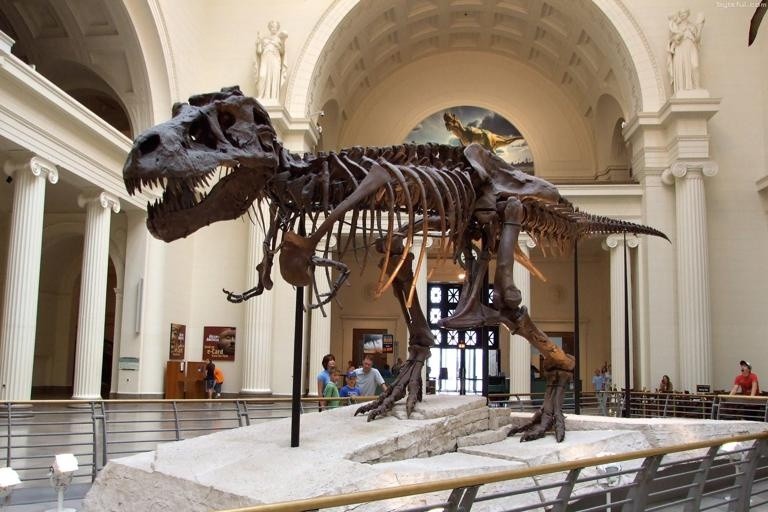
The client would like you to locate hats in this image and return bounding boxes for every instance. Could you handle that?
[348,372,356,377]
[740,360,752,369]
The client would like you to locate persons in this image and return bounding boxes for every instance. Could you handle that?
[171,324,182,351]
[591,361,613,416]
[659,376,675,392]
[205,358,215,398]
[217,328,235,355]
[531,364,541,382]
[318,354,403,411]
[214,368,224,398]
[730,360,762,396]
